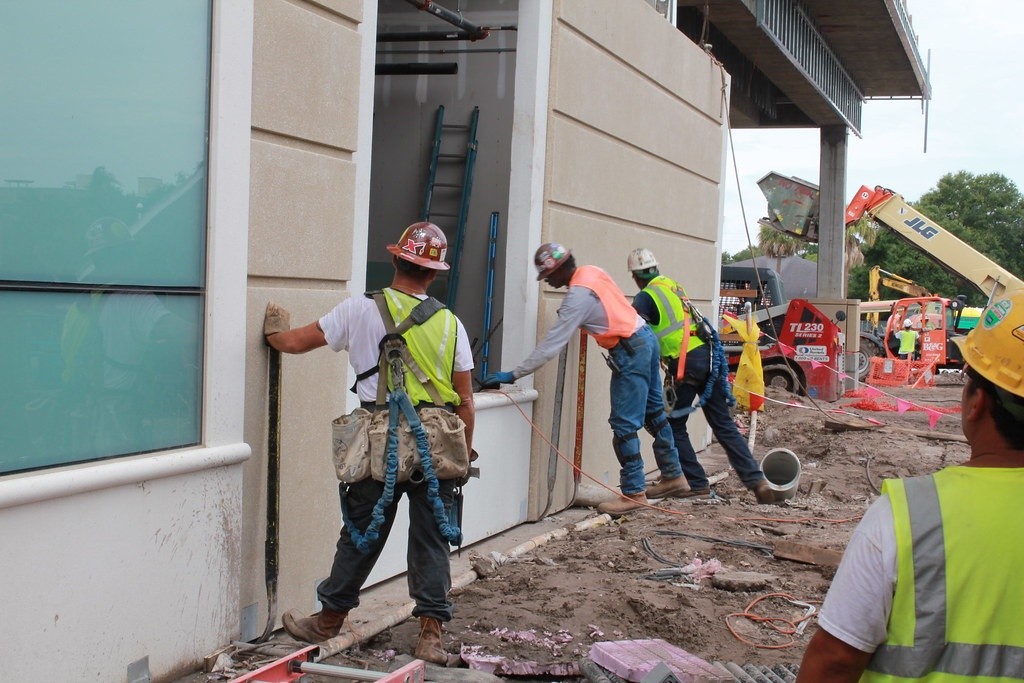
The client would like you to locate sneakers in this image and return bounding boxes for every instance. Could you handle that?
[679,484,711,497]
[756,480,775,504]
[599,491,647,513]
[645,473,688,500]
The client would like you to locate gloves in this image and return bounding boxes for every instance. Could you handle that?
[263,303,291,334]
[479,371,513,388]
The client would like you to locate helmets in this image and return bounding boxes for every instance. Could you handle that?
[902,318,912,328]
[920,315,929,321]
[629,249,659,270]
[385,222,450,270]
[534,242,572,282]
[951,289,1024,400]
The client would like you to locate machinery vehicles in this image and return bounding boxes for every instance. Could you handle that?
[753,171,1024,381]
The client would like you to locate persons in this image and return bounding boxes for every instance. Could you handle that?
[920,315,937,331]
[790,284,1024,683]
[479,241,692,518]
[626,247,777,507]
[893,319,921,361]
[261,219,478,667]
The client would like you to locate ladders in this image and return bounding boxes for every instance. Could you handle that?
[413,101,483,335]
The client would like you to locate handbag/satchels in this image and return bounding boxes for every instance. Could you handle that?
[327,404,468,481]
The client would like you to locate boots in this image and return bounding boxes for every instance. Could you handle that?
[418,615,448,664]
[283,605,344,647]
